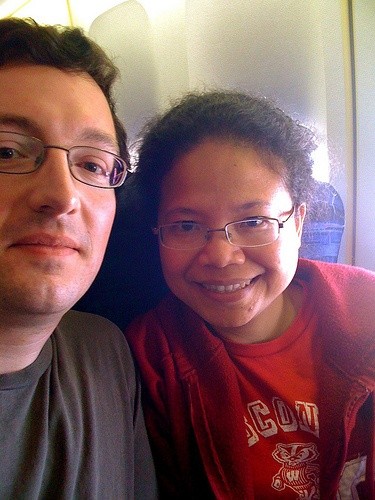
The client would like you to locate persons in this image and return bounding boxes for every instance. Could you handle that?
[0,18,159,500]
[124,90,375,500]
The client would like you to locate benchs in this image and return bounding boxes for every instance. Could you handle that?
[71,173,346,329]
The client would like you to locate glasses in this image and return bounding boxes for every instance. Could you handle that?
[0,130,129,188]
[152,201,296,251]
